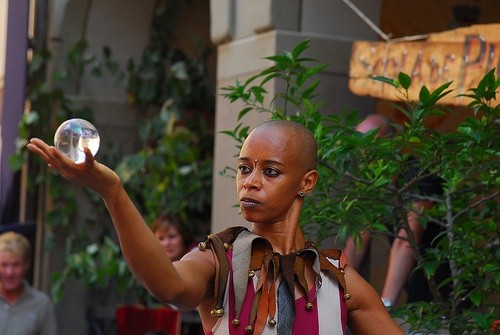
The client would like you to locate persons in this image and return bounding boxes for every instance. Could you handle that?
[0,232,57,335]
[152,212,198,257]
[27,119,406,335]
[338,115,479,311]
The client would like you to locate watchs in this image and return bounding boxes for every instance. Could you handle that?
[382,298,393,310]
